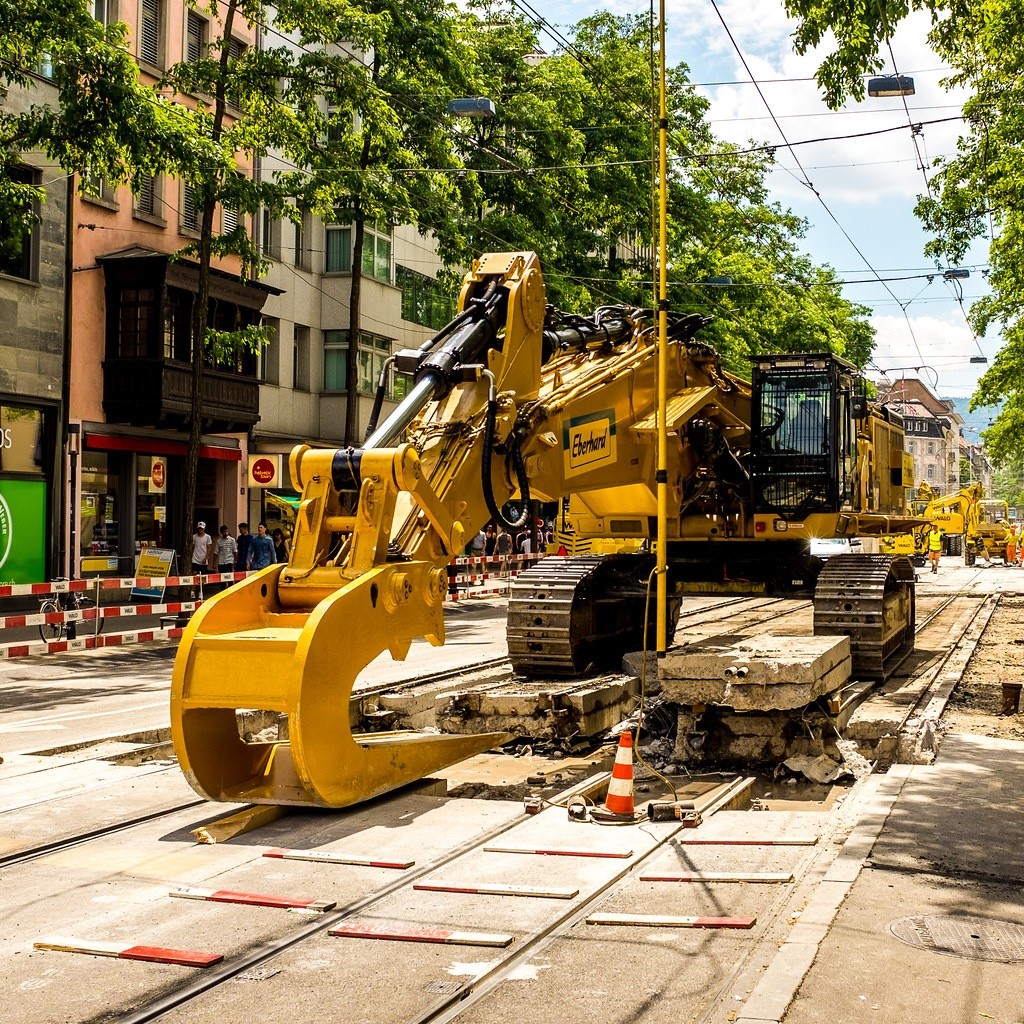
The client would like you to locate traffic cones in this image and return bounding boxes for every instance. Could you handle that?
[589,731,642,824]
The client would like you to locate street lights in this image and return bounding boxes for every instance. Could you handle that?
[66,421,81,639]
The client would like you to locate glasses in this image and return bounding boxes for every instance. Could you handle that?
[277,533,279,536]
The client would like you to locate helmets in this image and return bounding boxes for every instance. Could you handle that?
[1010,528,1015,534]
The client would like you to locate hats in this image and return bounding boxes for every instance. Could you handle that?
[197,522,206,528]
[259,522,269,534]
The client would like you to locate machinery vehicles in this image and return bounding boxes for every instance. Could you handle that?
[264,489,297,542]
[883,479,1018,567]
[170,251,931,809]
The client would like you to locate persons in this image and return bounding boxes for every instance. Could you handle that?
[1017,525,1024,568]
[467,525,555,586]
[190,521,212,600]
[212,522,291,590]
[1005,528,1020,567]
[928,525,945,575]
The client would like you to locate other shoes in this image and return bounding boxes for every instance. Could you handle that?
[199,592,203,598]
[190,592,195,598]
[1017,563,1022,567]
[933,570,937,574]
[930,565,935,572]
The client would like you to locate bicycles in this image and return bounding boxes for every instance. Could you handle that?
[39,576,104,643]
